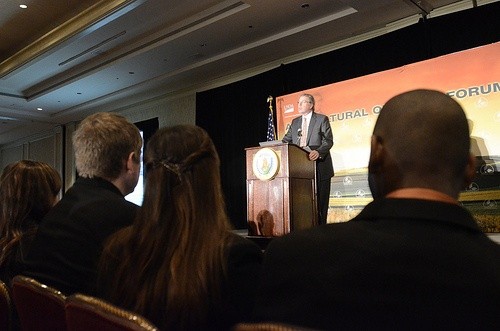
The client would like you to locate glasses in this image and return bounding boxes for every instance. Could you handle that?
[298,101,311,104]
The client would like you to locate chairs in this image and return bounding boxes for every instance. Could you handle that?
[0,275,314,331]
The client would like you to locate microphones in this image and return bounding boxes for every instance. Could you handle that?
[297,129,302,136]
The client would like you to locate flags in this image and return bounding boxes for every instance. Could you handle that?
[266,106,277,141]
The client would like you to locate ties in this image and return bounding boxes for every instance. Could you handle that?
[300,118,308,147]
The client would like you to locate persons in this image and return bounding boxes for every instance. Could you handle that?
[281,93,334,225]
[263,89,500,331]
[28,112,143,297]
[94,124,262,331]
[0,159,62,283]
